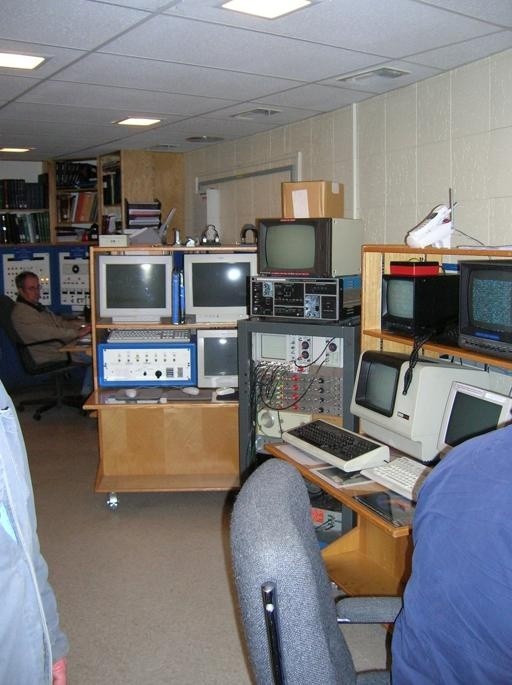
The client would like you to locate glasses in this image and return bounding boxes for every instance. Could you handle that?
[26,285,42,291]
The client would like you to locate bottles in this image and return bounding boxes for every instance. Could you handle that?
[83,223,98,241]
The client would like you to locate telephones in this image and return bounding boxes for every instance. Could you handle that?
[406,187,459,248]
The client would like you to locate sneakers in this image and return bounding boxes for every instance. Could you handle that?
[61,394,91,417]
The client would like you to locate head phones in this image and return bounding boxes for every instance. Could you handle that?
[241,226,259,245]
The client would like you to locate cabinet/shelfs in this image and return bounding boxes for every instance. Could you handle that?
[0,150,186,358]
[82,244,257,510]
[265,245,512,636]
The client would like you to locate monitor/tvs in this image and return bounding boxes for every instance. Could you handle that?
[258,218,364,278]
[197,329,238,396]
[184,254,258,323]
[99,256,173,325]
[349,349,491,463]
[437,381,512,454]
[458,260,512,362]
[381,275,460,341]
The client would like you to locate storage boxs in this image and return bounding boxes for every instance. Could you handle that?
[281,181,345,219]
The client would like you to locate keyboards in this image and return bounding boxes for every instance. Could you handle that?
[281,419,389,473]
[360,456,432,503]
[108,329,191,343]
[80,333,92,343]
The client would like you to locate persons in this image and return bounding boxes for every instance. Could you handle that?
[391,423,511,684]
[0,377,72,684]
[12,271,99,414]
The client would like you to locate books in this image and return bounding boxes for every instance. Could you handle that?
[1,160,97,243]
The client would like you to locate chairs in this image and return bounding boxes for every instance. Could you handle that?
[230,458,403,685]
[0,295,89,420]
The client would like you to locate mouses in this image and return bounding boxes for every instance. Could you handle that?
[181,387,199,396]
[125,388,137,399]
